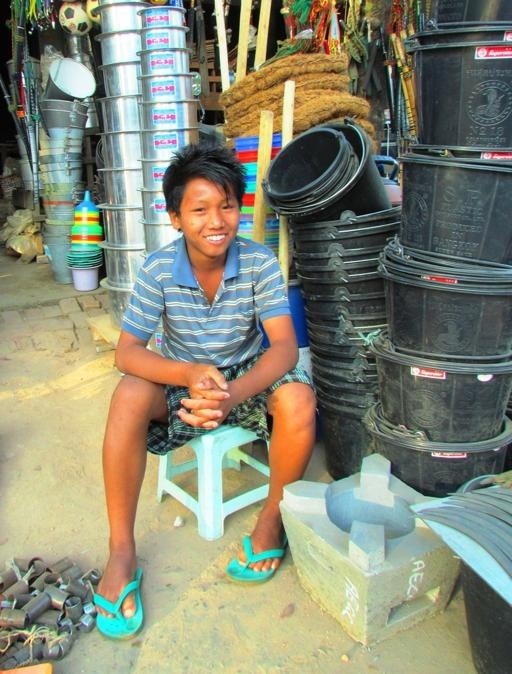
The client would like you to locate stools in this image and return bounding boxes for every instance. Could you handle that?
[155,424,273,542]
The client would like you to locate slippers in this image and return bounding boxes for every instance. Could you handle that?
[226,532,289,586]
[95,567,143,642]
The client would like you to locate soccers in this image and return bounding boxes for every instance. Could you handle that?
[59,1,92,36]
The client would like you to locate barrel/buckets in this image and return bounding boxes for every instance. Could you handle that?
[42,53,88,285]
[264,119,389,477]
[94,2,152,313]
[141,9,200,258]
[69,189,102,294]
[379,4,511,493]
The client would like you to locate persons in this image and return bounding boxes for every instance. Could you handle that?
[92,138,318,643]
